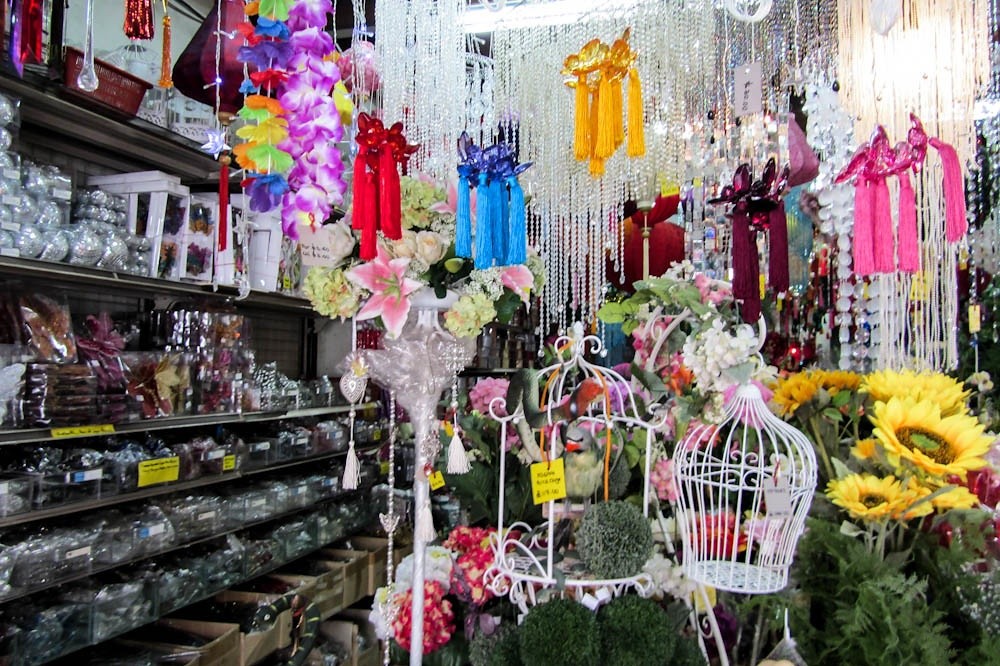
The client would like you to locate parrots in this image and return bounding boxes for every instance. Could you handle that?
[560,425,607,517]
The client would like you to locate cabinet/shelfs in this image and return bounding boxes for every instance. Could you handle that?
[0,81,388,666]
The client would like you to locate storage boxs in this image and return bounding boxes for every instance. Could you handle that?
[116,523,413,666]
[63,46,154,115]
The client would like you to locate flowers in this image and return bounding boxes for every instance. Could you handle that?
[221,174,1000,666]
